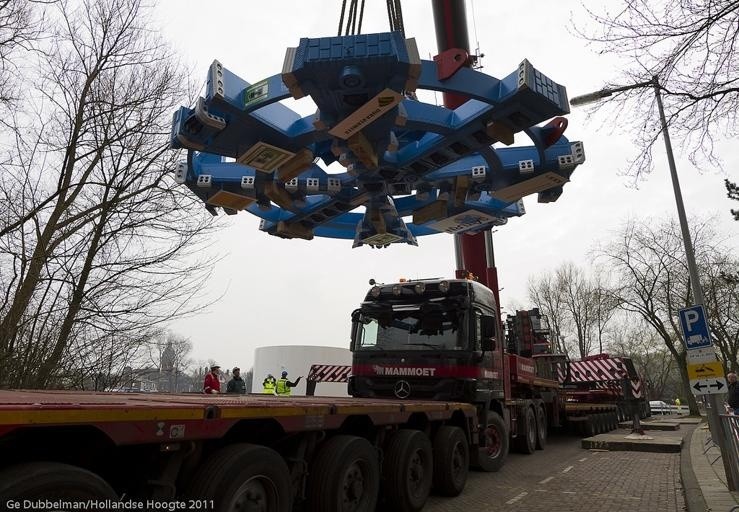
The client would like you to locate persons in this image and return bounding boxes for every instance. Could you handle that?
[226,367,246,394]
[263,374,276,394]
[727,372,739,425]
[274,371,304,396]
[204,363,220,393]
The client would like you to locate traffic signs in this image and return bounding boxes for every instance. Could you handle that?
[685,346,729,395]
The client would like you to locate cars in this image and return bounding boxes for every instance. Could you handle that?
[649,400,672,415]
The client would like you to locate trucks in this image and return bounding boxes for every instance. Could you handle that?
[346,266,626,478]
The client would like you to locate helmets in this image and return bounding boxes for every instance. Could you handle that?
[233,367,240,373]
[282,371,288,375]
